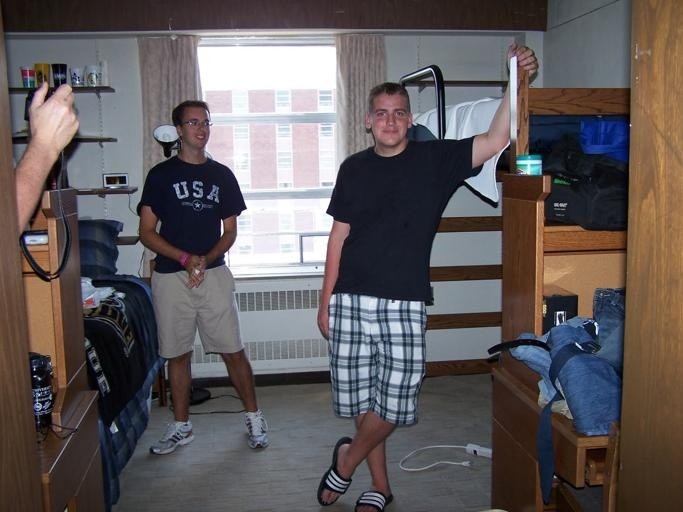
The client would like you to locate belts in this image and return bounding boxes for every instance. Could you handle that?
[488,338,551,354]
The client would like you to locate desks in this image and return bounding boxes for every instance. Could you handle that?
[483,356,628,511]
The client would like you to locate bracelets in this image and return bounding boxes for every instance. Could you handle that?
[180,251,191,266]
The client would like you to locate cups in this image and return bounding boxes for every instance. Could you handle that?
[16,63,99,88]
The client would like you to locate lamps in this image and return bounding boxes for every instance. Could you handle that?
[153,125,180,159]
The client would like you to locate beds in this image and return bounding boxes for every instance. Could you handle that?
[81,274,167,506]
[399,55,629,355]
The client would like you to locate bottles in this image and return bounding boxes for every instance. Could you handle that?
[515,155,542,176]
[27,354,53,430]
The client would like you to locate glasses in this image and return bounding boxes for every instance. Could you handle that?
[182,121,213,128]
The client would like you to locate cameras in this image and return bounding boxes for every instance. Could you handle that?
[25,90,54,121]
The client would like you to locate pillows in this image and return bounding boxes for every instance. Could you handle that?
[79,220,123,281]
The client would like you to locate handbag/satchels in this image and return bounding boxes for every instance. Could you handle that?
[527,132,628,231]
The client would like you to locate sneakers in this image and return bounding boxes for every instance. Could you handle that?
[150,421,194,454]
[244,409,271,449]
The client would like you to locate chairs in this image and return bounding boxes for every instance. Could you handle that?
[555,422,619,511]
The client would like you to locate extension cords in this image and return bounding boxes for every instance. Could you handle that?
[466,443,492,459]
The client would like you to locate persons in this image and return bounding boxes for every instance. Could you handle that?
[136,99,270,456]
[16,80,80,238]
[317,40,541,511]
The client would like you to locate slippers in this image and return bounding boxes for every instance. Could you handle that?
[355,491,393,512]
[318,437,353,506]
